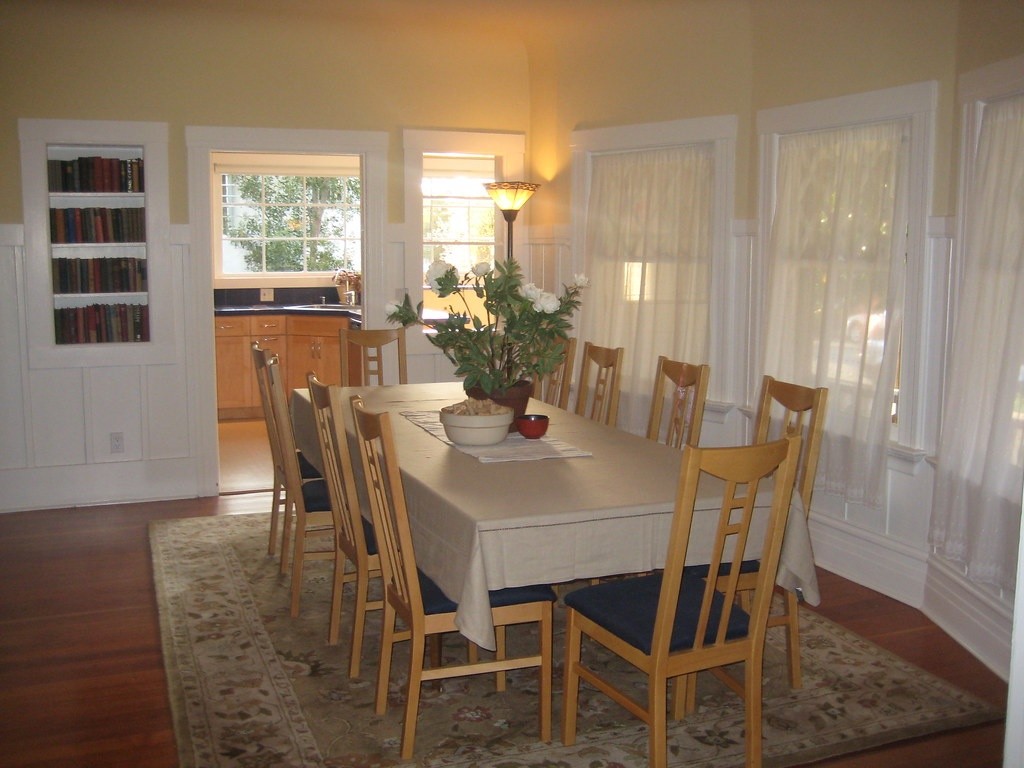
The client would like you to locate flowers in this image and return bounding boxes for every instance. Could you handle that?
[386,259,590,391]
[331,271,363,290]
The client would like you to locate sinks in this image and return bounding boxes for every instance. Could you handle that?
[297,305,362,309]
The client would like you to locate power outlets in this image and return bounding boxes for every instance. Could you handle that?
[260,288,275,302]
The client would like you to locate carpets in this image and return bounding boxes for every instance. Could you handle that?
[148,510,1008,768]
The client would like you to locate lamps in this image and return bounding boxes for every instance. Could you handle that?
[483,181,539,265]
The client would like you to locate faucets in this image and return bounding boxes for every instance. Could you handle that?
[335,269,356,306]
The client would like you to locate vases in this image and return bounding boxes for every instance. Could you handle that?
[336,284,359,305]
[463,375,536,432]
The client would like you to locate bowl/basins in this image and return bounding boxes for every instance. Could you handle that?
[517,414,549,441]
[440,412,514,445]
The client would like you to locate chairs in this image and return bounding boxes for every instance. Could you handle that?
[591,356,712,585]
[653,375,829,691]
[251,339,287,555]
[307,367,403,679]
[266,351,332,618]
[571,341,625,426]
[351,396,555,757]
[339,326,406,389]
[562,434,800,768]
[525,331,577,409]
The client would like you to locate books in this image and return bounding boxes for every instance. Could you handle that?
[54,303,150,345]
[49,208,146,244]
[52,257,148,294]
[48,157,145,193]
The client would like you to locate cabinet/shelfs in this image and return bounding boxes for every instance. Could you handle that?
[215,313,362,422]
[49,192,149,297]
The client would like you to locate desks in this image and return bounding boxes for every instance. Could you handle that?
[289,381,820,691]
